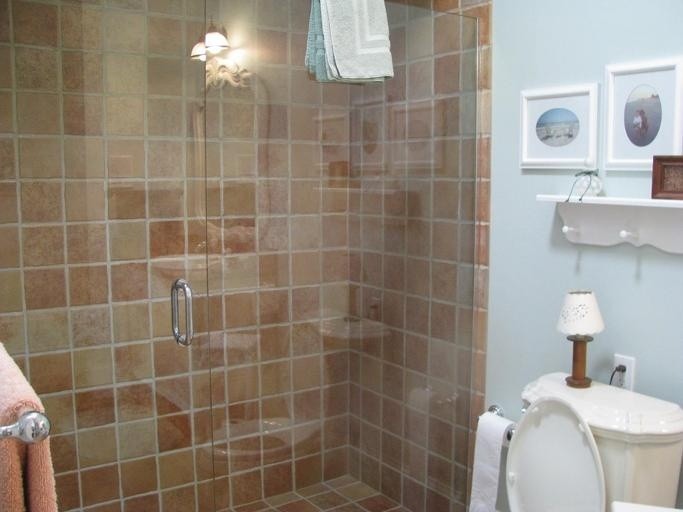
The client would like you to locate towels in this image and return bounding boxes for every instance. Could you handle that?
[0,341,59,512]
[302,0,395,86]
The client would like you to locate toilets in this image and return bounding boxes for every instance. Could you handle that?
[197,311,394,477]
[506,372,683,512]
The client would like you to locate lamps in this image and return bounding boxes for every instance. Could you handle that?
[191,32,230,61]
[557,291,605,388]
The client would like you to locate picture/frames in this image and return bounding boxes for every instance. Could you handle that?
[519,57,683,200]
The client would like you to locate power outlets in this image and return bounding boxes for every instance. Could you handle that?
[612,353,635,392]
